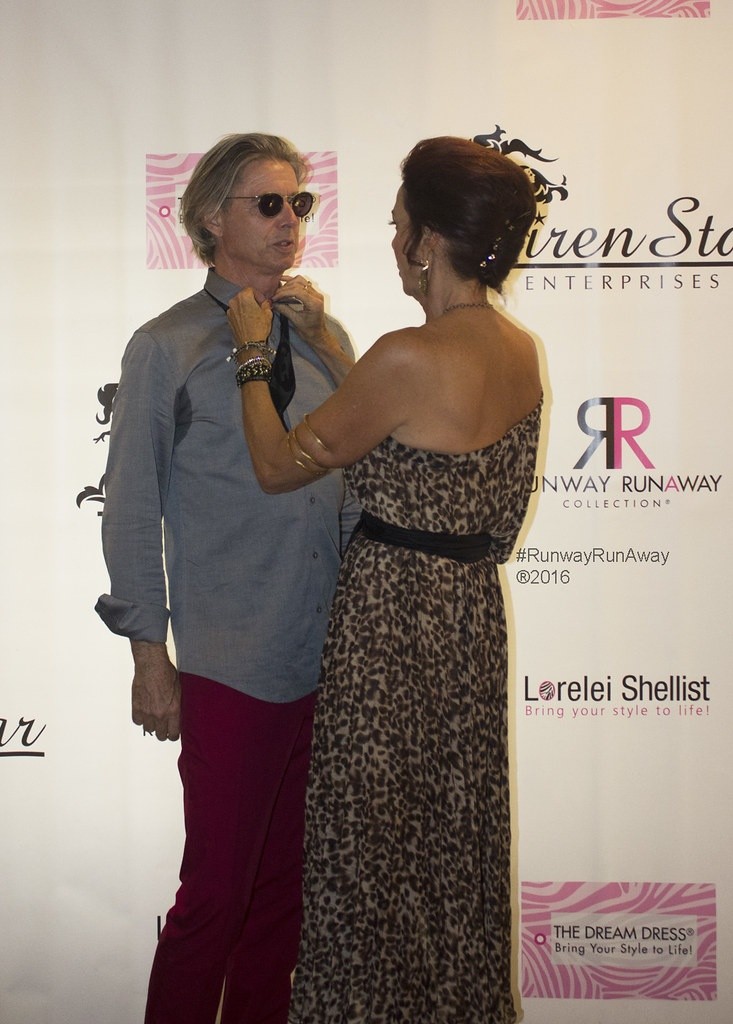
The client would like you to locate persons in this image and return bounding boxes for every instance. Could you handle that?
[94,132,364,1024]
[228,135,544,1024]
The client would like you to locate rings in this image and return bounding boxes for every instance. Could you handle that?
[304,285,308,290]
[307,281,312,287]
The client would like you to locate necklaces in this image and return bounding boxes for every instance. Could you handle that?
[443,303,494,314]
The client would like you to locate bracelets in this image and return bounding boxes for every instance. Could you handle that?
[226,340,277,388]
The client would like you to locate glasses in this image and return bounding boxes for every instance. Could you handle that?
[226,192,313,217]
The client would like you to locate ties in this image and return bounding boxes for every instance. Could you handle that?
[204,287,296,434]
